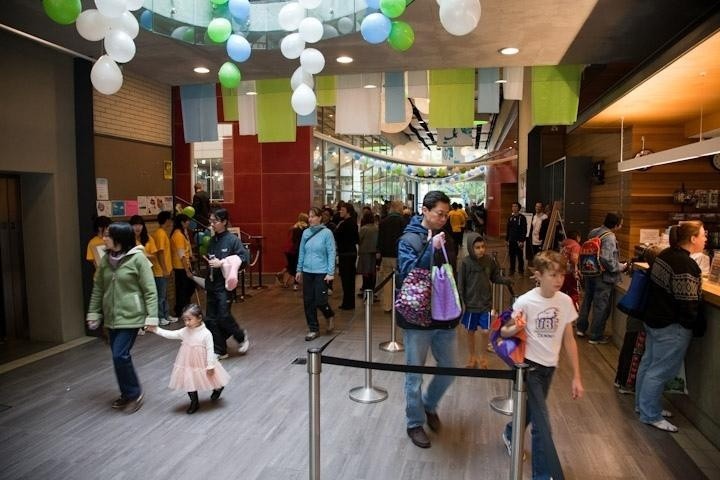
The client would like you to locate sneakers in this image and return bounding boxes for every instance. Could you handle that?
[325,317,335,332]
[588,335,612,344]
[305,331,319,341]
[576,329,585,337]
[113,316,248,416]
[614,382,635,395]
[502,432,526,461]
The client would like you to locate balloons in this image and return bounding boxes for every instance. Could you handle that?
[278,1,326,118]
[168,25,187,39]
[182,29,195,44]
[337,18,356,34]
[357,12,393,45]
[389,21,418,52]
[208,1,254,91]
[347,149,486,185]
[362,0,381,11]
[322,23,339,42]
[438,0,482,36]
[44,0,83,26]
[381,1,410,19]
[74,0,145,97]
[139,10,153,31]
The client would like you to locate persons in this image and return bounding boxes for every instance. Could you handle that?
[612,243,664,395]
[86,221,161,416]
[85,180,232,335]
[560,211,628,344]
[395,190,461,449]
[457,232,514,369]
[142,302,231,414]
[505,201,564,276]
[446,201,488,255]
[201,207,250,360]
[499,249,584,480]
[632,220,710,434]
[282,198,417,342]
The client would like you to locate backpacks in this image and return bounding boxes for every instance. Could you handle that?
[578,232,615,278]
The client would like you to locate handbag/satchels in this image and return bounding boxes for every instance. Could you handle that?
[488,307,527,369]
[616,268,649,319]
[393,263,462,329]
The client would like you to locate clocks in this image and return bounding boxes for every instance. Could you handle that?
[711,153,720,171]
[633,148,654,171]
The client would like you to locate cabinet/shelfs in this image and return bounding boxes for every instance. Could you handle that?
[526,156,591,255]
[526,123,569,210]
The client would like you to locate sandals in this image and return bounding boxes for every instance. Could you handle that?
[661,410,673,417]
[652,419,678,433]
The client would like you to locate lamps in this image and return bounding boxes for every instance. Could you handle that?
[617,108,720,172]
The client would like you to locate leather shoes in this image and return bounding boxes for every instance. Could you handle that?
[425,410,440,432]
[407,427,431,448]
